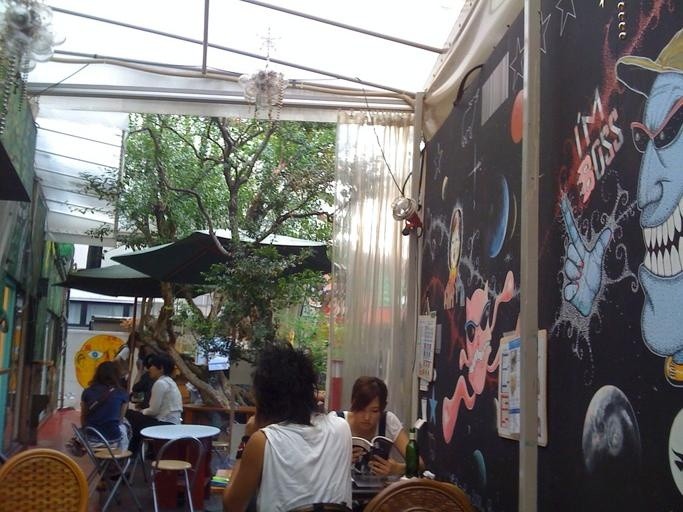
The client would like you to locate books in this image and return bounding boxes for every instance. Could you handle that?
[351,435,393,482]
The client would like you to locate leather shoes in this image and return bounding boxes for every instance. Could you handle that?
[111,473,130,480]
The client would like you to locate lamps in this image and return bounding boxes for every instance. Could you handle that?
[237,23,288,134]
[391,193,424,238]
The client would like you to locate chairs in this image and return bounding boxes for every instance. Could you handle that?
[289,502,350,511]
[363,477,472,510]
[83,427,146,510]
[2,451,87,510]
[150,435,202,511]
[72,426,128,505]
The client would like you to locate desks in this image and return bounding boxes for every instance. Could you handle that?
[349,473,407,501]
[140,423,222,497]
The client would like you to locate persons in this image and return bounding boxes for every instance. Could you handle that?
[114,331,140,391]
[95,355,182,490]
[326,376,425,477]
[80,361,132,474]
[222,339,352,511]
[132,352,159,410]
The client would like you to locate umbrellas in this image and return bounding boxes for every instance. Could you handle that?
[51,264,221,394]
[109,227,331,290]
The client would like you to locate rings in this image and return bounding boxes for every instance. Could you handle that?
[382,464,384,469]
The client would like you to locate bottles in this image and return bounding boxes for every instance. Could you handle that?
[405,427,419,479]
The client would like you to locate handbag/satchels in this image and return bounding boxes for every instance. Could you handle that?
[65,437,87,457]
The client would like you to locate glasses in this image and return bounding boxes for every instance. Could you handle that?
[629,97,682,153]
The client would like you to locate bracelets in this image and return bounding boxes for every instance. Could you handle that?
[235,435,250,458]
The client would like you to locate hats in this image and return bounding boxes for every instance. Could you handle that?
[611,28,682,97]
